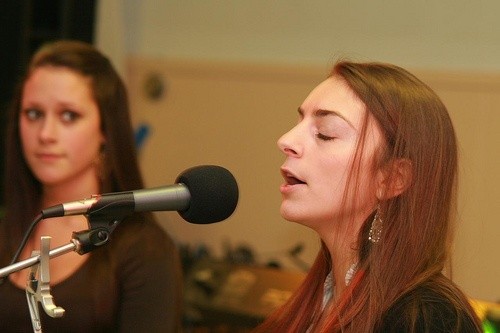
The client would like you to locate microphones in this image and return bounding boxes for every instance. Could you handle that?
[40,164,238,226]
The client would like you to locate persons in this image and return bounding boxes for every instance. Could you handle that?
[0,38,186,333]
[248,62,482,333]
